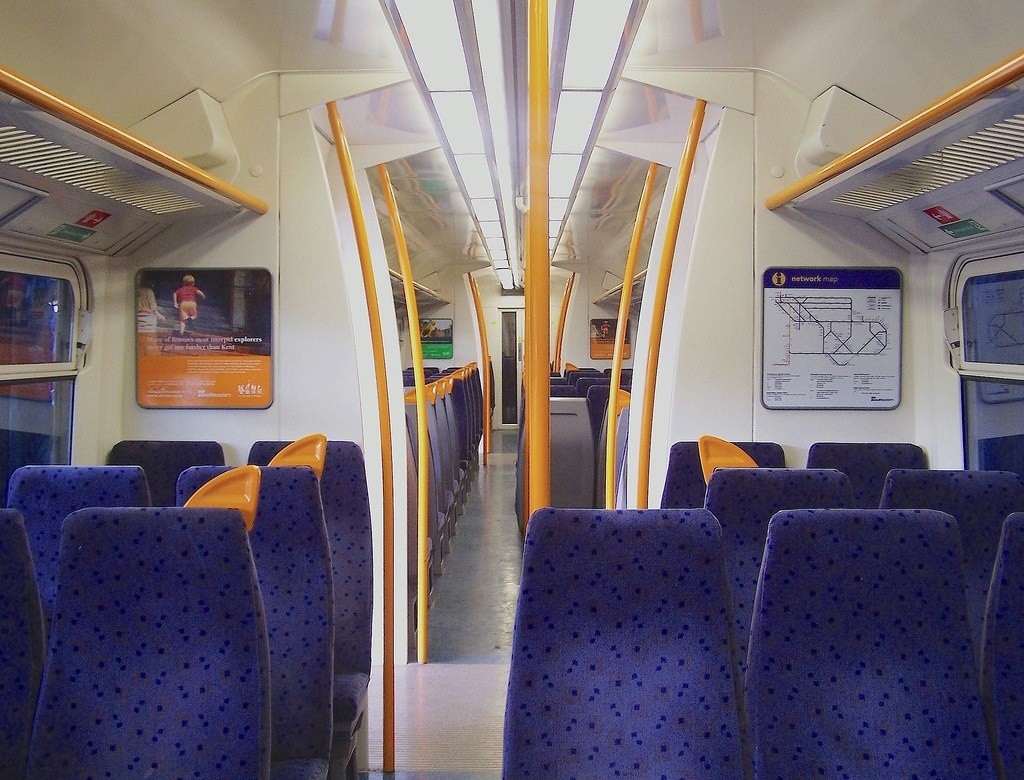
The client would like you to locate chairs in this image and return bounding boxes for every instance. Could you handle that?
[514,367,1024,780]
[0,359,495,780]
